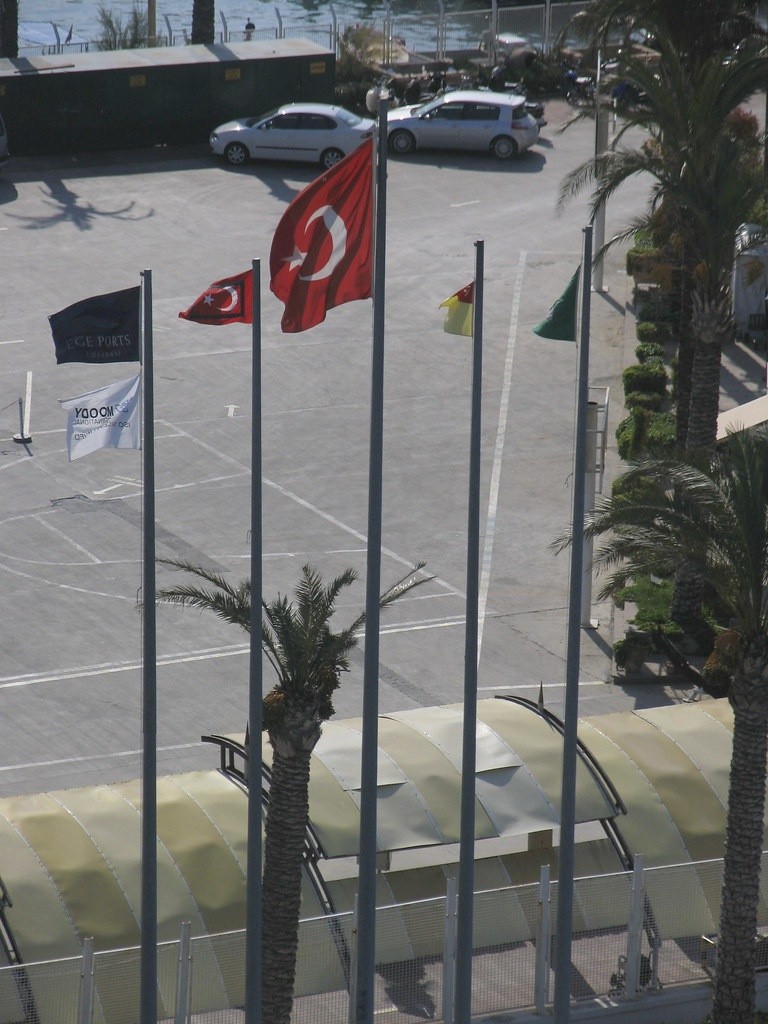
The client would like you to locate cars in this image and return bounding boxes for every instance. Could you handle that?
[383,88,539,162]
[206,99,378,173]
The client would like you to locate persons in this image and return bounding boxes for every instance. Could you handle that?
[366,74,448,115]
[245,17,256,29]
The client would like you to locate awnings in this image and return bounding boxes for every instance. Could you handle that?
[0,694,768,1024]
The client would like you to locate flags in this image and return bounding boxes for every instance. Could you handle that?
[46,286,142,366]
[179,269,253,326]
[56,371,142,462]
[270,136,373,333]
[437,281,475,339]
